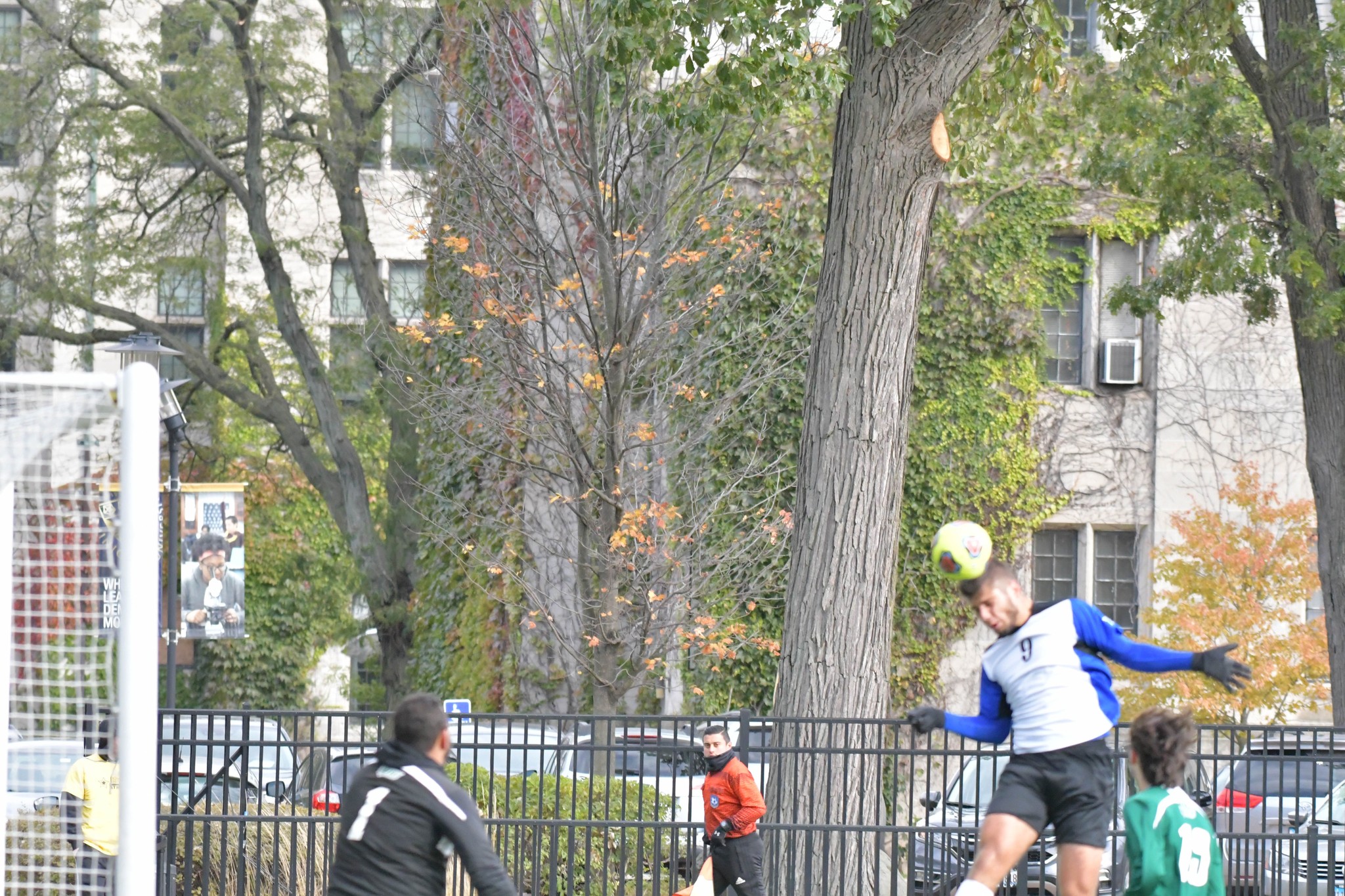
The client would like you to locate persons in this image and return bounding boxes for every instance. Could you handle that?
[68,720,122,896]
[907,559,1252,896]
[699,724,768,896]
[183,513,246,626]
[325,692,519,896]
[1126,708,1225,896]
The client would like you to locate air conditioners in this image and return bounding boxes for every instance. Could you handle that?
[1100,339,1139,384]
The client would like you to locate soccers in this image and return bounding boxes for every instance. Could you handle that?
[930,520,993,581]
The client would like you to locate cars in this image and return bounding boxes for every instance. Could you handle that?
[1260,777,1344,896]
[6,706,781,874]
[1209,723,1345,887]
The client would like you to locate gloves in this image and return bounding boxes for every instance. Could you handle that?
[1193,643,1251,695]
[702,834,710,845]
[711,819,734,847]
[908,707,943,734]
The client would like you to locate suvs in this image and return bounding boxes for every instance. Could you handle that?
[907,741,1210,895]
